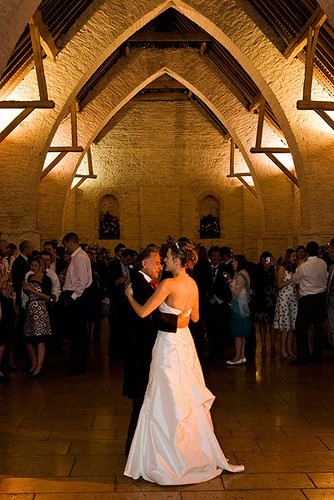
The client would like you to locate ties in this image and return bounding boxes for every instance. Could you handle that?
[148,281,158,289]
[210,266,218,279]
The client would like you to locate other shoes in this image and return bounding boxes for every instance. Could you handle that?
[290,358,305,364]
[23,368,35,376]
[261,350,268,356]
[306,355,320,363]
[0,372,9,384]
[271,351,277,356]
[289,352,296,359]
[7,364,18,371]
[124,439,133,456]
[279,352,292,359]
[29,366,44,378]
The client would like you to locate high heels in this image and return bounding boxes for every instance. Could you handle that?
[226,359,243,366]
[242,357,247,366]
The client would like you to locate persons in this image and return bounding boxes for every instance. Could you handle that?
[121,246,190,454]
[0,232,334,378]
[123,238,245,485]
